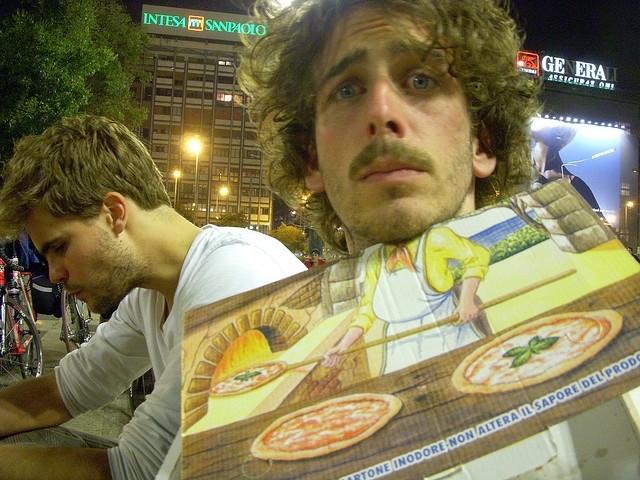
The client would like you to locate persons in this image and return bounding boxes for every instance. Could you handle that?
[628,247,640,263]
[235,0,535,257]
[1,114,313,479]
[304,249,327,268]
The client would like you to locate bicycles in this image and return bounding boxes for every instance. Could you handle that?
[0,247,42,389]
[60,286,92,353]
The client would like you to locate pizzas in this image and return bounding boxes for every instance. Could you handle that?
[211,362,286,397]
[452,309,626,399]
[249,391,403,462]
